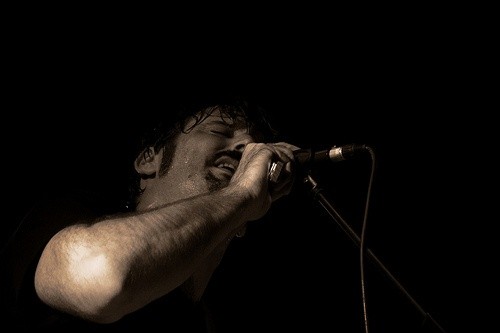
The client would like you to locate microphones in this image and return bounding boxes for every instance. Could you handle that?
[270,141,367,164]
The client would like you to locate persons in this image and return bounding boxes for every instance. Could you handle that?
[32,90,301,333]
